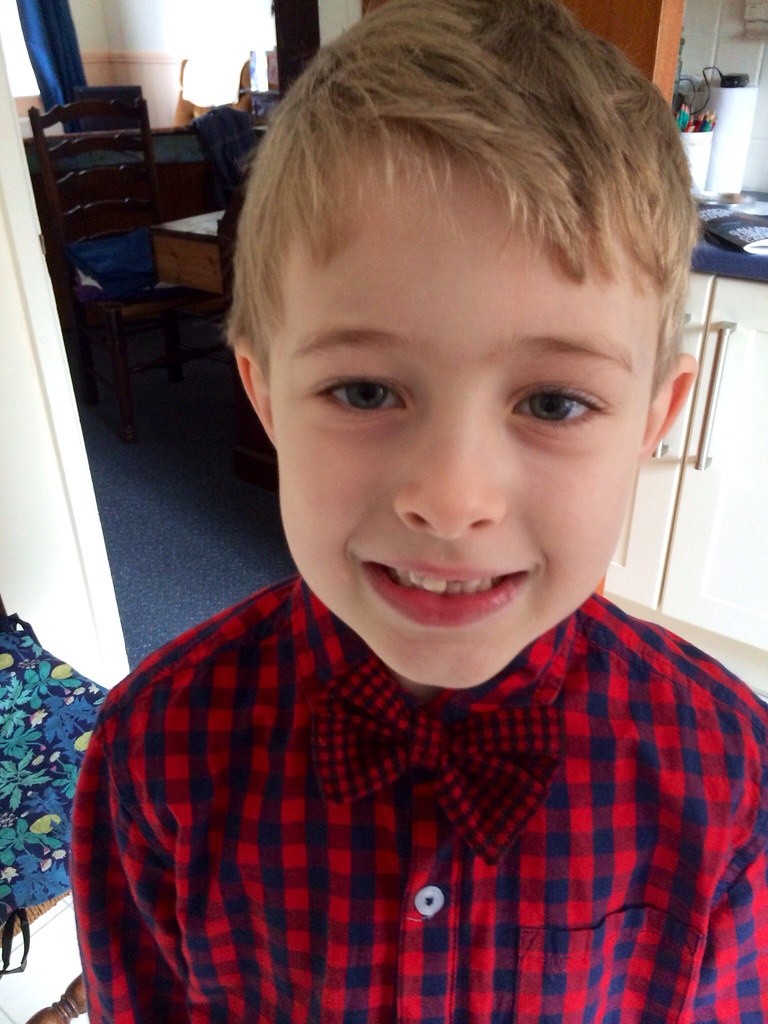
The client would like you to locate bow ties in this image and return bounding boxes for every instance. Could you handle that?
[319,653,558,865]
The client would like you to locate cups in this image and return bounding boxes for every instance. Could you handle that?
[680,132,713,192]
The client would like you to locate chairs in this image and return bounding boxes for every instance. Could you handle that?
[170,55,251,124]
[27,98,228,446]
[72,83,148,130]
[1,598,111,1024]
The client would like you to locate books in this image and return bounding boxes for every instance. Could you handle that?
[698,205,768,255]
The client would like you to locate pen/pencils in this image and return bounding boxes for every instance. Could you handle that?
[675,102,717,133]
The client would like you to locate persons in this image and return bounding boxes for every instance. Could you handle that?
[69,2,768,1024]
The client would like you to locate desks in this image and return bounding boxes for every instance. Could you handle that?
[148,203,223,295]
[22,127,217,329]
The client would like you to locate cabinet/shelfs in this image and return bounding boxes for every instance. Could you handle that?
[602,275,768,661]
